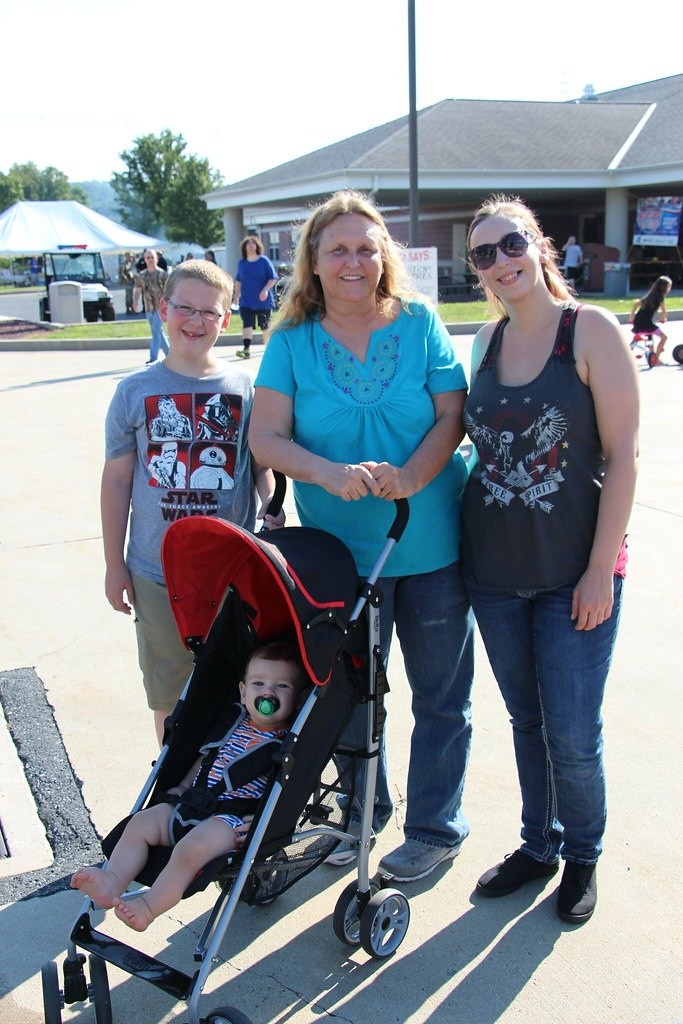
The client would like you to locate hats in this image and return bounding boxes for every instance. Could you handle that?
[125,252,133,257]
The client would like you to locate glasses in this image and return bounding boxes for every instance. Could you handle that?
[467,230,538,271]
[164,298,228,322]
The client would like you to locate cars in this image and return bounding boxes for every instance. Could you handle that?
[0,269,28,285]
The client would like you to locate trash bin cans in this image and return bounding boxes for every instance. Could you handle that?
[604,262,632,298]
[48,281,84,324]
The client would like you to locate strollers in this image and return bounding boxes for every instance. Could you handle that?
[40,467,409,1024]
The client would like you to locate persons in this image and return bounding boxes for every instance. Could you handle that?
[101,260,287,747]
[29,255,40,285]
[205,250,217,264]
[70,641,299,932]
[562,236,583,288]
[133,249,169,364]
[235,237,278,358]
[248,192,472,879]
[459,204,642,922]
[121,251,193,314]
[630,276,672,365]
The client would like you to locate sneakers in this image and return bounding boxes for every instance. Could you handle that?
[376,839,463,881]
[321,818,376,866]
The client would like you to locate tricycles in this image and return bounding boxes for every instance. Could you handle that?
[673,344,683,364]
[625,320,665,367]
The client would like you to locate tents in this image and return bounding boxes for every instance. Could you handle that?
[0,199,167,298]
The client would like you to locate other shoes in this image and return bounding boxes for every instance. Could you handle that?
[126,310,131,314]
[236,348,250,360]
[131,310,140,315]
[557,858,597,923]
[146,360,156,366]
[476,850,559,897]
